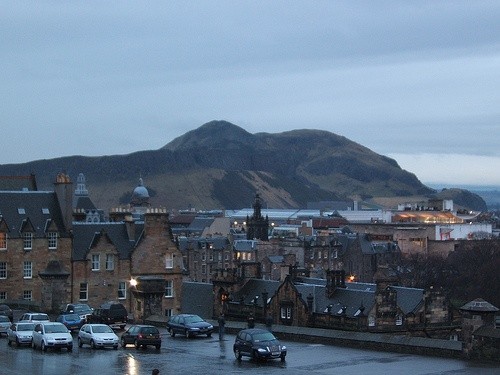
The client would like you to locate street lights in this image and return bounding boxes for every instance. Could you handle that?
[261,288,269,323]
[129,278,138,313]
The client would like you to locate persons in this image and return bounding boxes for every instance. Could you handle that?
[152,369,160,375]
[218,313,226,341]
[246,311,256,329]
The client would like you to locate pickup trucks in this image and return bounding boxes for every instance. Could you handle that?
[61,303,95,322]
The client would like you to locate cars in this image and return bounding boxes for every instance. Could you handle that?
[0,316,13,337]
[55,314,81,329]
[166,313,214,337]
[233,328,287,362]
[32,321,73,350]
[78,323,119,349]
[7,322,36,346]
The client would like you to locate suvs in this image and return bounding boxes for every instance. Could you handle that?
[120,324,162,349]
[93,303,129,329]
[18,313,50,324]
[0,305,14,322]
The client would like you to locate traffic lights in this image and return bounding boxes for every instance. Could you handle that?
[220,293,229,301]
